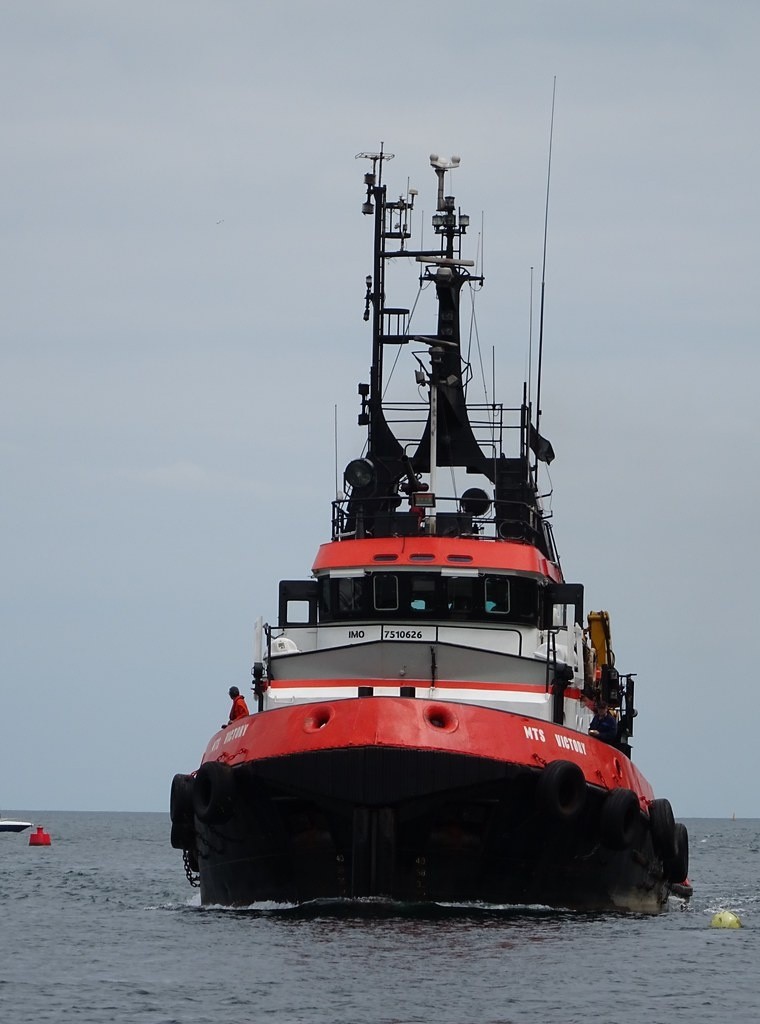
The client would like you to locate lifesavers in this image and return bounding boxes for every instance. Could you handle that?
[586,647,598,689]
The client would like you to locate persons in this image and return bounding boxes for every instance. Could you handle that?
[587,700,618,742]
[221,686,248,727]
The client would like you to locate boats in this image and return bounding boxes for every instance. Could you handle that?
[166,62,699,918]
[0,818,31,832]
